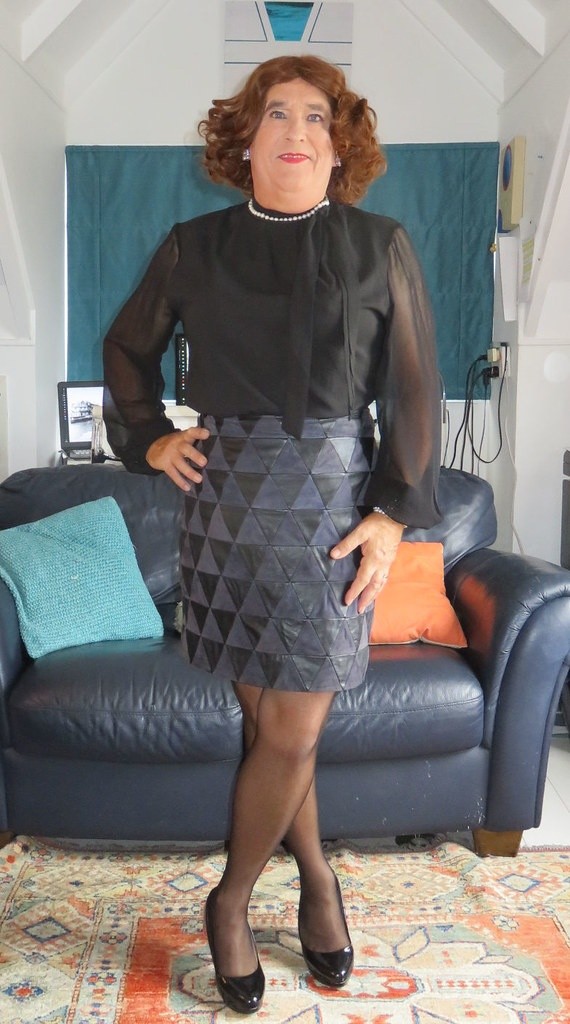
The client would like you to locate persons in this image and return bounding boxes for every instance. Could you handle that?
[101,54,442,1014]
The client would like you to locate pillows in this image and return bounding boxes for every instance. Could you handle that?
[0,495,166,660]
[365,541,470,653]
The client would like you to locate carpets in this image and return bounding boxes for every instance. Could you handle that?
[0,831,570,1024]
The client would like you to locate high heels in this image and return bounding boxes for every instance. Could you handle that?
[204,886,266,1014]
[297,873,353,988]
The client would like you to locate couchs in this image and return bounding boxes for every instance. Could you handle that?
[0,463,570,858]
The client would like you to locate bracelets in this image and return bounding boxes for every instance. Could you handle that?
[373,506,408,528]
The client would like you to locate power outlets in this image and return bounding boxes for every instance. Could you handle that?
[489,343,511,379]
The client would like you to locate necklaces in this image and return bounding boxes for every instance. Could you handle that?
[248,194,330,221]
